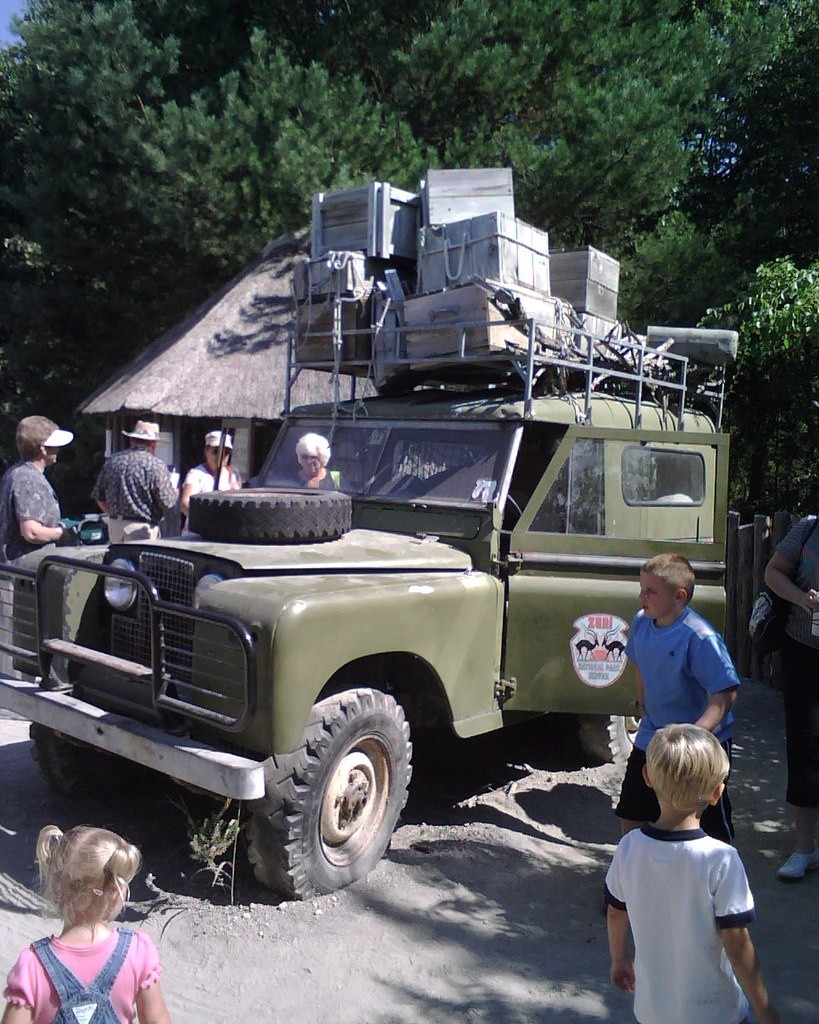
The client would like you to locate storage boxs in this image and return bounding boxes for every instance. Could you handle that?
[290,166,623,380]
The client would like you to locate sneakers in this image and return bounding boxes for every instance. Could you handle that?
[777,840,819,878]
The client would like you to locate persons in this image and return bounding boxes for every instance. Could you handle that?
[0,825,171,1024]
[614,553,740,844]
[91,420,179,544]
[765,515,819,879]
[0,416,80,564]
[288,433,347,492]
[603,724,782,1024]
[180,431,242,536]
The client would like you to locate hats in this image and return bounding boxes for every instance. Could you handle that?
[205,431,233,450]
[41,429,74,446]
[122,420,168,442]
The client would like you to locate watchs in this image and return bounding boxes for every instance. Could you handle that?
[635,699,645,710]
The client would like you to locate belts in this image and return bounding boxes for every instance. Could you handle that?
[110,514,157,525]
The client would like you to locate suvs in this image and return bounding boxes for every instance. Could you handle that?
[0,322,729,899]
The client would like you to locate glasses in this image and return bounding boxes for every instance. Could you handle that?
[208,448,230,456]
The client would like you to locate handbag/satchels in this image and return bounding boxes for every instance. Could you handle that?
[56,513,109,546]
[748,515,819,653]
